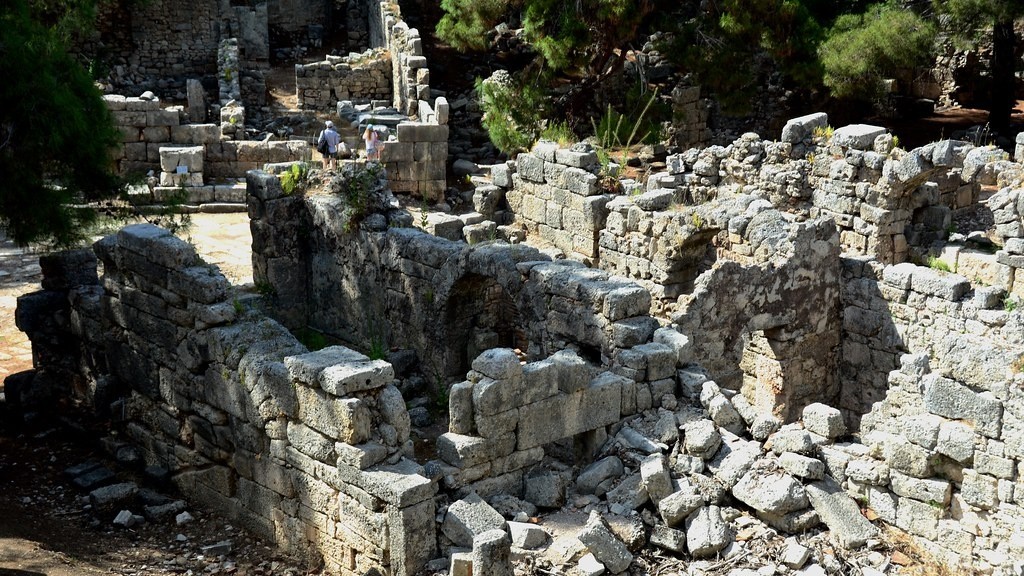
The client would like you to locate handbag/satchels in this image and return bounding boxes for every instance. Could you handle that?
[317,131,328,154]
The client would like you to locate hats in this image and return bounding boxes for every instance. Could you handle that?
[325,121,333,127]
[367,124,373,131]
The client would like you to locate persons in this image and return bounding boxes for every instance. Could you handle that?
[362,124,377,160]
[317,120,338,172]
